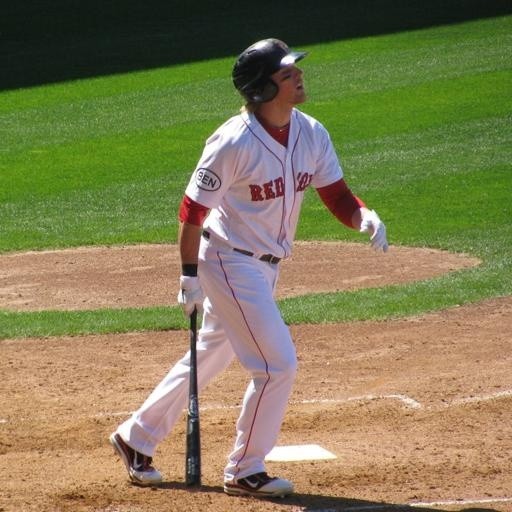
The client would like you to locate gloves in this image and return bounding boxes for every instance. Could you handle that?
[176,273,204,318]
[357,209,389,254]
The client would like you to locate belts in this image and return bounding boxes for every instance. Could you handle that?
[203,230,281,265]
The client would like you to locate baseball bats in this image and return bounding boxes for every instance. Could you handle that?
[186,308,200,491]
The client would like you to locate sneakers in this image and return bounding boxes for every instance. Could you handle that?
[224,470,295,498]
[110,431,163,487]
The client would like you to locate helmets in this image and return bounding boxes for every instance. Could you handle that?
[231,38,310,103]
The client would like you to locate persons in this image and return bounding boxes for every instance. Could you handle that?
[109,38,390,498]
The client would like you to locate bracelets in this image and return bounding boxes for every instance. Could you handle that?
[182,263,198,277]
